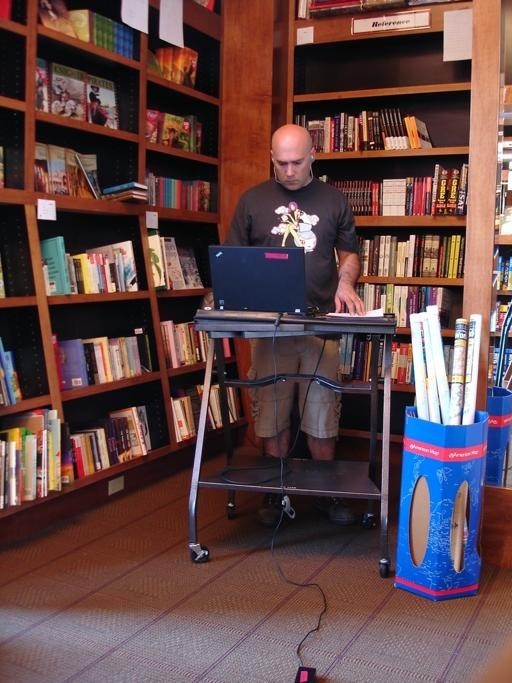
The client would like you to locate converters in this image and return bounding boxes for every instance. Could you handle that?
[294,666,317,683]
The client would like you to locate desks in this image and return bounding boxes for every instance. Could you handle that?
[188,305,406,580]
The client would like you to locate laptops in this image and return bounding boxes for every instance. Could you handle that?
[207,245,307,313]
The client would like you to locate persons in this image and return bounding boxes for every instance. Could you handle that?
[222,124,366,526]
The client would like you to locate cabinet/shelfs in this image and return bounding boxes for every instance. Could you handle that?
[285,2,511,448]
[2,2,250,544]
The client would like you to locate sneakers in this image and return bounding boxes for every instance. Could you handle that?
[258,493,279,525]
[314,496,357,525]
